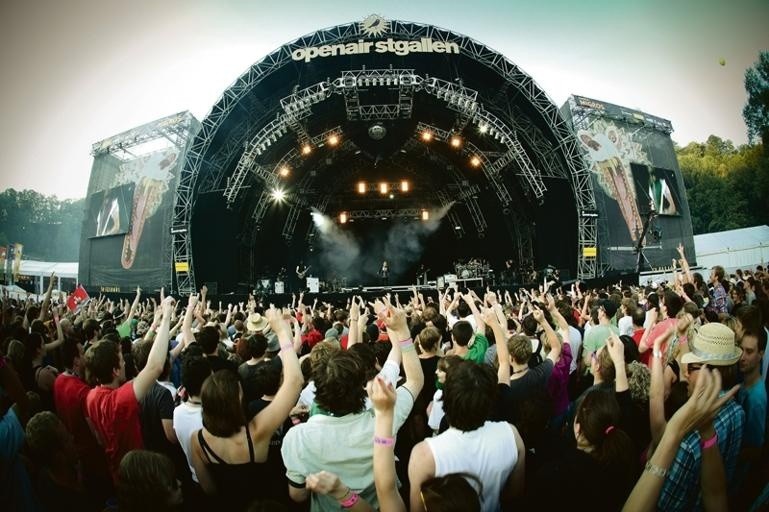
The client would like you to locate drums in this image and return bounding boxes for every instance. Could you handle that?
[462,270,473,278]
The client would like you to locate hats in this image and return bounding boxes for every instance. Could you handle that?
[681,322,743,366]
[247,313,268,331]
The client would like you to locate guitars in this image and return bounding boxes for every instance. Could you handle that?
[298,265,312,279]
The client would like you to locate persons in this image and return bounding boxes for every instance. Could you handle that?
[93,190,119,236]
[647,165,676,214]
[2,241,768,512]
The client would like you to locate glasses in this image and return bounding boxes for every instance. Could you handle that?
[687,364,700,374]
[591,350,597,360]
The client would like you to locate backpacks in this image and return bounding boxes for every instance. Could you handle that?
[527,339,544,370]
